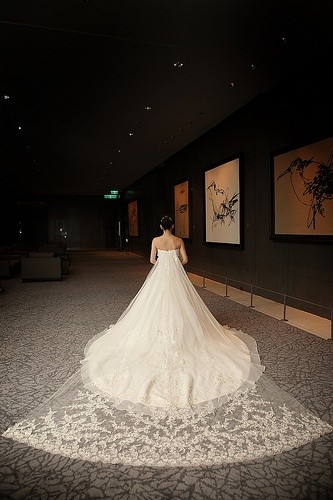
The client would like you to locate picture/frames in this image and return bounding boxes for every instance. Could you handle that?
[203,156,244,250]
[128,199,139,238]
[173,180,191,240]
[269,137,333,243]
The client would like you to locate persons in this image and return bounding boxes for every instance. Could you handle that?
[149,214,188,356]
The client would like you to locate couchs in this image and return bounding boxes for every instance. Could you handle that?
[0,239,71,283]
